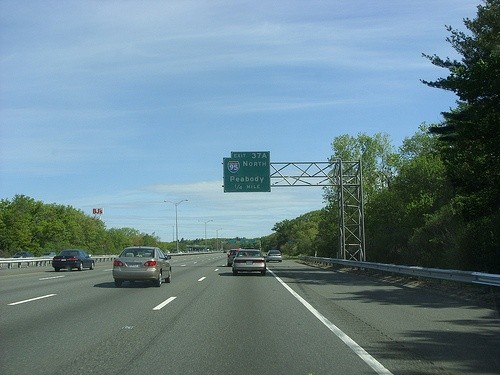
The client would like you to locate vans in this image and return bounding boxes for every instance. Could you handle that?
[11,251,33,259]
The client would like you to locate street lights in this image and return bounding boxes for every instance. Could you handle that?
[163,199,189,253]
[198,220,214,251]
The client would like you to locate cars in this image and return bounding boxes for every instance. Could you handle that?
[112,247,173,288]
[227,247,268,276]
[40,252,56,257]
[266,250,283,262]
[52,250,94,272]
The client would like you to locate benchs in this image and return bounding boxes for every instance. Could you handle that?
[126,253,152,257]
